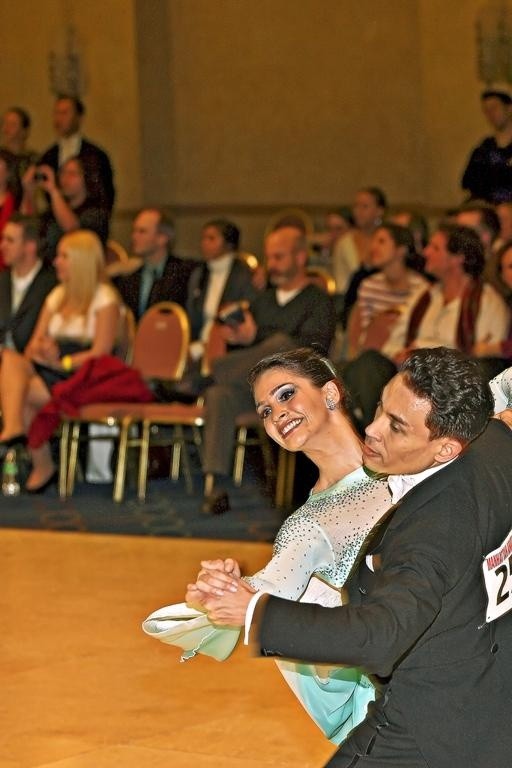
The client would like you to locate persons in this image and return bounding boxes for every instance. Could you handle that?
[1,212,127,496]
[460,89,512,204]
[113,185,512,515]
[1,146,109,264]
[1,89,116,210]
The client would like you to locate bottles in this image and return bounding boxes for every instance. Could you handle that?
[2,448,19,496]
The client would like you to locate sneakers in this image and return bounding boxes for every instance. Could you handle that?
[143,460,170,480]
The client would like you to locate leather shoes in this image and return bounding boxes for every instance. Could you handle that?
[149,375,202,407]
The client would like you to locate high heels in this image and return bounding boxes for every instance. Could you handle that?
[22,459,61,498]
[0,430,28,450]
